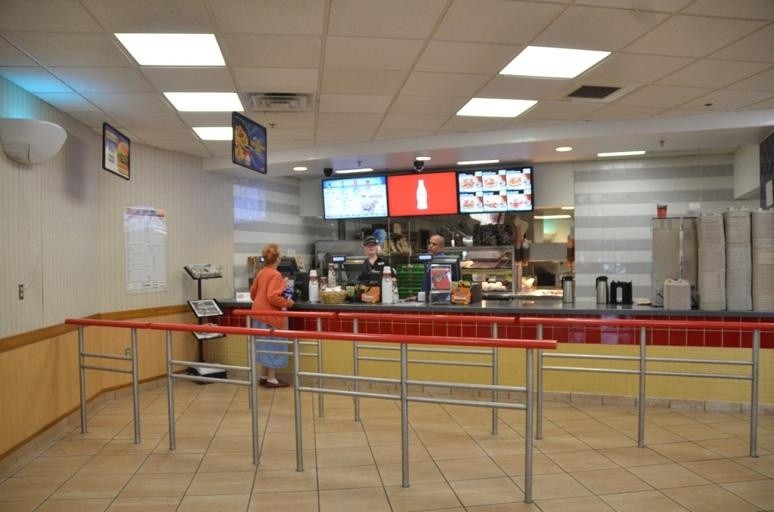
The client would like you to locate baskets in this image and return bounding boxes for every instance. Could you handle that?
[319,288,347,305]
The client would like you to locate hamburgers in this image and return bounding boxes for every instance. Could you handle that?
[117,142,128,176]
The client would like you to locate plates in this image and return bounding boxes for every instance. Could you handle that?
[459,172,532,211]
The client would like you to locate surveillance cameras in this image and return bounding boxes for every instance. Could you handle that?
[413,161,424,172]
[323,168,333,177]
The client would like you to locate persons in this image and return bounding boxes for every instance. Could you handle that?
[355,236,395,287]
[404,235,457,302]
[249,244,295,389]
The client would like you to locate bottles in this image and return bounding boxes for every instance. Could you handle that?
[665,279,691,311]
[561,276,574,303]
[248,256,262,290]
[594,276,608,304]
[415,179,428,209]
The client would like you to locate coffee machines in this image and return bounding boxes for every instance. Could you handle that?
[326,252,402,287]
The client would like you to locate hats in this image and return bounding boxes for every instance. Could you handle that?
[362,236,379,245]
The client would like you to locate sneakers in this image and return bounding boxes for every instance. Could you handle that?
[260,377,290,388]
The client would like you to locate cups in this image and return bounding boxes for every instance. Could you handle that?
[417,292,425,302]
[307,264,336,303]
[381,265,400,305]
[656,203,667,219]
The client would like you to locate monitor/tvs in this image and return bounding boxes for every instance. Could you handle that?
[278,259,300,274]
[344,256,369,280]
[431,256,463,282]
